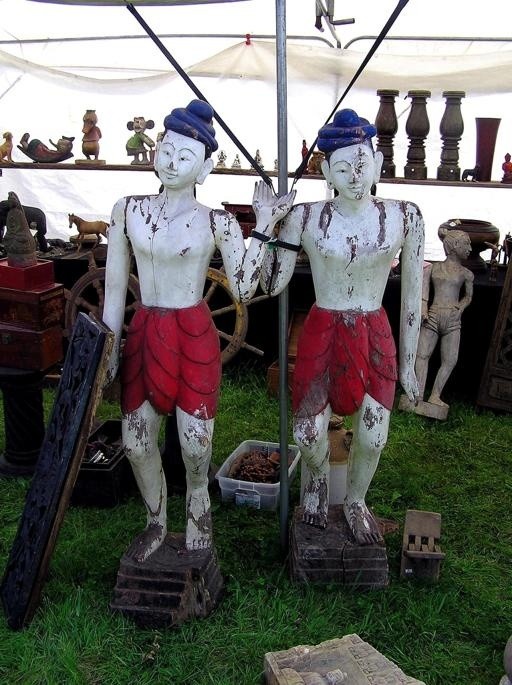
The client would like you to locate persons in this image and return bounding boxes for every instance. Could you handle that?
[416,224,482,408]
[249,105,428,549]
[1,189,37,256]
[98,97,301,569]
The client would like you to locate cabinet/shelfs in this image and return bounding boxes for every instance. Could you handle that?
[0,160,511,415]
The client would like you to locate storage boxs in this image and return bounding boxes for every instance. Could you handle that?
[0,256,56,292]
[221,201,257,238]
[0,282,66,332]
[215,439,303,513]
[265,307,312,399]
[68,417,138,509]
[0,325,65,373]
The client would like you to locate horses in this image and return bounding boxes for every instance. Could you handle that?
[68,213,110,251]
